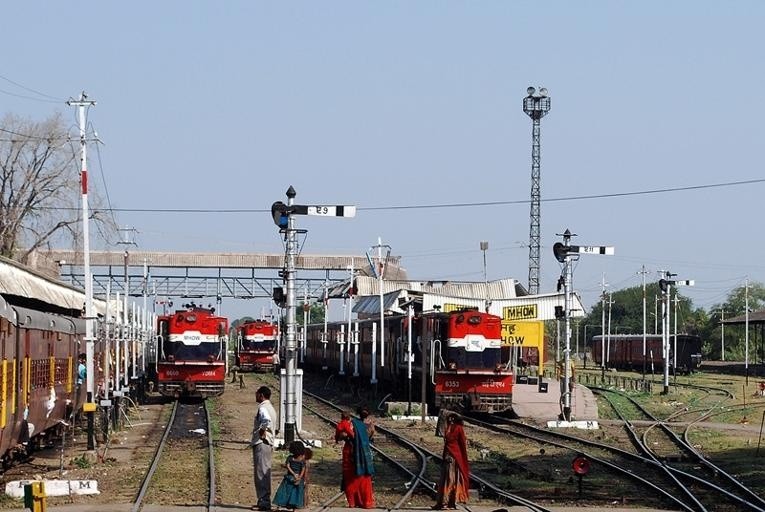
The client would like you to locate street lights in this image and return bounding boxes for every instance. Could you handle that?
[521,86,553,298]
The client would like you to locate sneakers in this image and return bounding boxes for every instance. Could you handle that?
[429,502,459,511]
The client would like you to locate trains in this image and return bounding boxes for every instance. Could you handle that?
[0,287,151,466]
[591,330,704,377]
[147,292,230,404]
[280,303,517,423]
[227,315,280,373]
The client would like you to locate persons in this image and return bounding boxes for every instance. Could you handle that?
[340,403,376,510]
[271,441,307,512]
[558,354,577,403]
[76,346,142,420]
[335,410,355,445]
[522,354,528,375]
[303,447,313,508]
[248,385,277,511]
[430,406,470,510]
[272,350,280,374]
